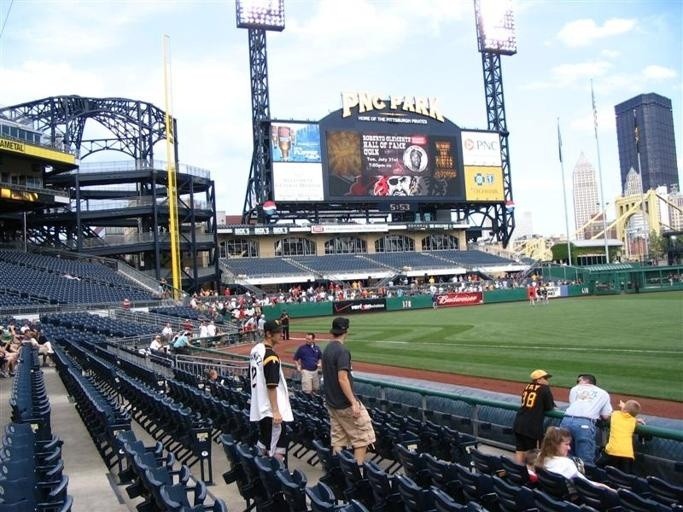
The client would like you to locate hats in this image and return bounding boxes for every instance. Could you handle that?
[530,369,552,381]
[264,319,288,333]
[330,318,347,335]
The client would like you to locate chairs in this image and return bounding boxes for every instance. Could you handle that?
[0,367,682,512]
[220,249,562,298]
[0,248,240,368]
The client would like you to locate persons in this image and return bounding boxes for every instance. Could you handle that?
[123,263,290,381]
[407,150,422,169]
[245,318,292,473]
[596,397,646,471]
[560,373,612,470]
[289,269,583,311]
[292,332,322,395]
[1,320,54,378]
[512,369,565,466]
[535,423,620,495]
[320,317,377,475]
[349,174,367,196]
[594,271,682,296]
[523,450,540,483]
